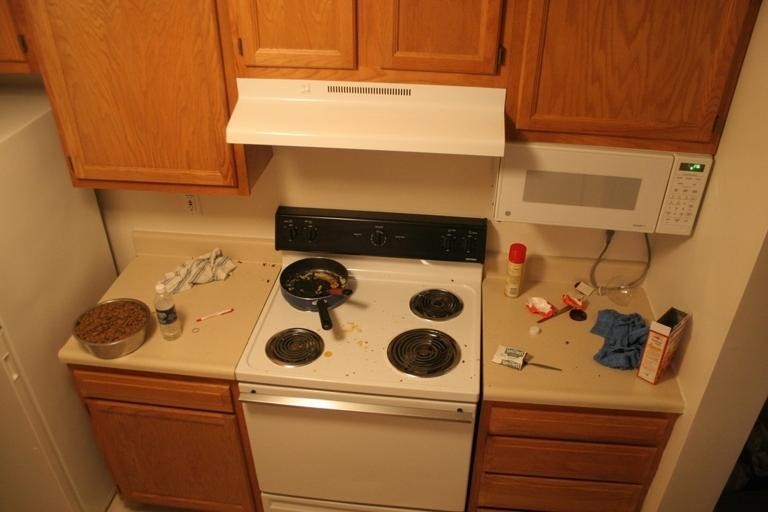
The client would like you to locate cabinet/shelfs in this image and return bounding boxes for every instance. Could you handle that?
[503,0,763,156]
[230,0,515,88]
[20,2,273,196]
[470,405,678,512]
[66,370,264,512]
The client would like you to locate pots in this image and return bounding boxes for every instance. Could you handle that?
[280,258,348,330]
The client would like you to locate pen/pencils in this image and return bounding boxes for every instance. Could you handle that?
[197,308,233,322]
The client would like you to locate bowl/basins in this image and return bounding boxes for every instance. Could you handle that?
[72,298,150,360]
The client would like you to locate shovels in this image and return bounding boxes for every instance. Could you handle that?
[312,282,352,298]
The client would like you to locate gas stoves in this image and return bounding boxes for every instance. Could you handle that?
[234,256,483,402]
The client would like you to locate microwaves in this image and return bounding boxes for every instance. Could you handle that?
[492,141,713,236]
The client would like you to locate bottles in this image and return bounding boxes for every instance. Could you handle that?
[154,284,182,341]
[504,243,527,297]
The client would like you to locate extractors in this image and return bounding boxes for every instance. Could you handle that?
[225,77,507,157]
